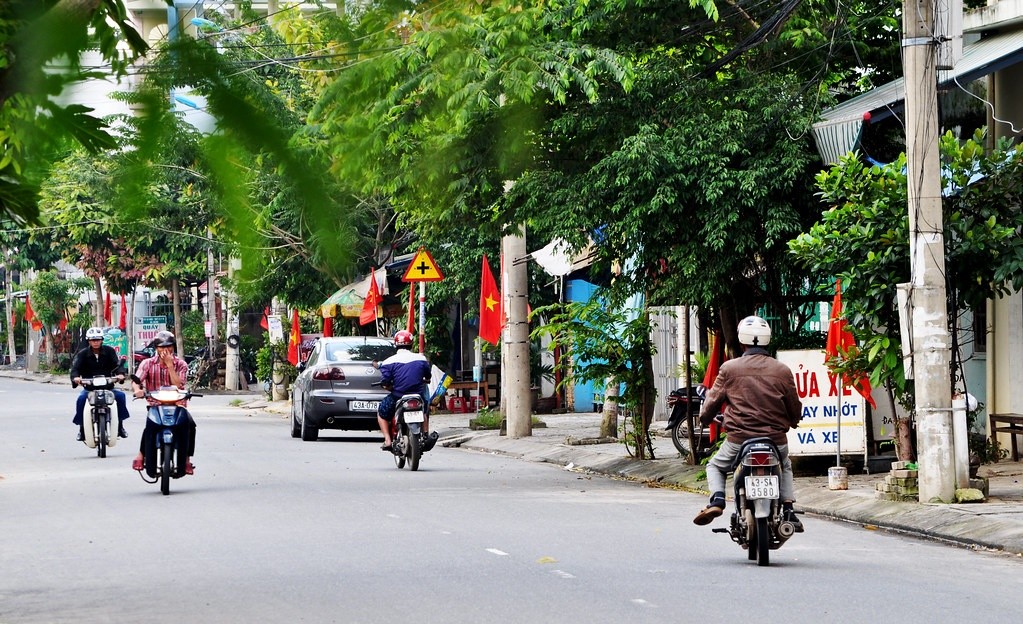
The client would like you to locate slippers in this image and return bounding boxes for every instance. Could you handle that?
[132,459,144,470]
[380,442,392,450]
[185,462,195,474]
[790,519,804,532]
[693,506,723,526]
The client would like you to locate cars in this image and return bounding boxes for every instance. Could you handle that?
[288,335,398,441]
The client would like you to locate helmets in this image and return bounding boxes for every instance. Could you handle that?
[151,331,175,347]
[393,330,414,346]
[85,327,104,341]
[736,316,771,346]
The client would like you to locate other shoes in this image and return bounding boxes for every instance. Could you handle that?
[76,432,85,441]
[119,427,129,439]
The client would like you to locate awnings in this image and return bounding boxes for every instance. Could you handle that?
[805,24,1022,172]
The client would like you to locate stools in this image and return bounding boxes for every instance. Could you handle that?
[449,397,468,413]
[470,396,484,413]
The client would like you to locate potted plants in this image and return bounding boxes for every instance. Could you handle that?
[530,330,541,411]
[969,449,981,479]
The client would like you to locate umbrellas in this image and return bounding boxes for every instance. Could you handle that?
[318,279,384,338]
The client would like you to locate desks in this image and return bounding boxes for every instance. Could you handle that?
[989,412,1023,463]
[448,380,489,412]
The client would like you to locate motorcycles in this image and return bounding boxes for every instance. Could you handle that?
[130,373,203,495]
[71,358,127,459]
[664,385,725,460]
[697,384,806,567]
[371,358,438,471]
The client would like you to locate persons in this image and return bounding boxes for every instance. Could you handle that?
[72,327,129,441]
[693,315,805,532]
[131,330,196,475]
[377,330,432,450]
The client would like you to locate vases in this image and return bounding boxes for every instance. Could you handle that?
[536,397,558,414]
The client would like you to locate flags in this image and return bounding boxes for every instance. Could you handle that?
[104,293,112,325]
[10,311,16,328]
[260,306,270,330]
[119,290,128,330]
[701,326,728,443]
[478,254,502,348]
[825,279,878,411]
[54,302,67,330]
[357,267,383,326]
[288,307,303,368]
[24,291,44,331]
